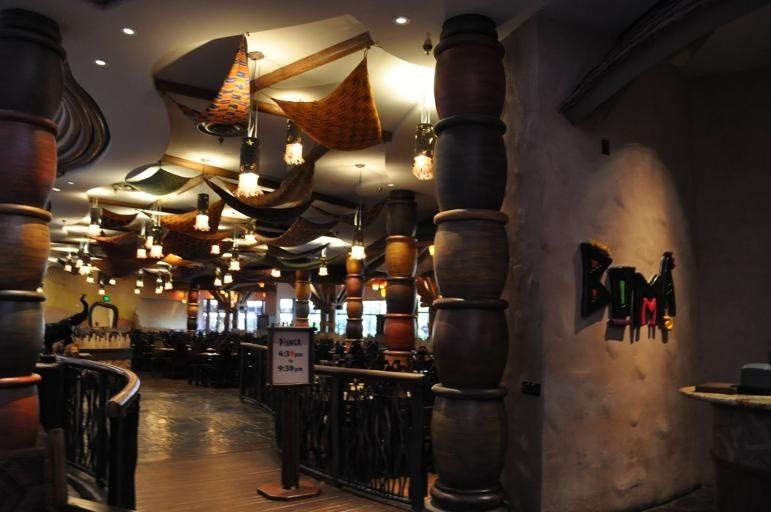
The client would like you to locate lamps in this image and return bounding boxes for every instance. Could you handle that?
[349,164,368,260]
[61,207,439,309]
[193,158,211,230]
[412,42,435,180]
[284,119,305,165]
[87,198,102,234]
[236,50,262,196]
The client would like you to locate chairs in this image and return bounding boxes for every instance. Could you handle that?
[300,334,438,496]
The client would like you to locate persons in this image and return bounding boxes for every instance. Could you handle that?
[382,360,407,372]
